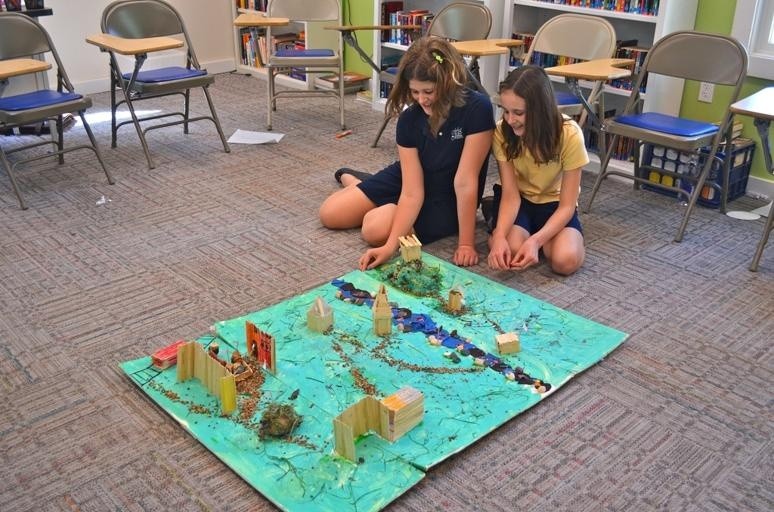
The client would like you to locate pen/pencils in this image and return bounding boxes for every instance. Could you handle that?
[336,129,353,138]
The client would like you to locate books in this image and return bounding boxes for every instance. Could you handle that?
[239,26,306,81]
[569,106,638,163]
[236,0,268,12]
[381,2,435,46]
[709,118,754,150]
[508,33,651,93]
[538,1,660,16]
[380,54,402,98]
[315,71,370,89]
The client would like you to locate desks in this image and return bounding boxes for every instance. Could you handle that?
[0,57,51,85]
[735,82,774,176]
[235,10,291,28]
[450,35,528,74]
[86,31,182,92]
[324,25,421,71]
[541,57,636,189]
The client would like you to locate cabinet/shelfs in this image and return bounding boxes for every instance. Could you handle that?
[502,0,699,184]
[231,0,337,98]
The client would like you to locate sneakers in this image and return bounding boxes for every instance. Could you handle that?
[481,195,497,223]
[335,168,375,183]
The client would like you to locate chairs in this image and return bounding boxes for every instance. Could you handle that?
[369,3,495,149]
[102,0,231,169]
[265,0,347,135]
[583,29,750,243]
[523,12,618,183]
[0,14,117,210]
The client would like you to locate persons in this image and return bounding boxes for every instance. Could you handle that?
[484,65,589,276]
[318,36,497,272]
